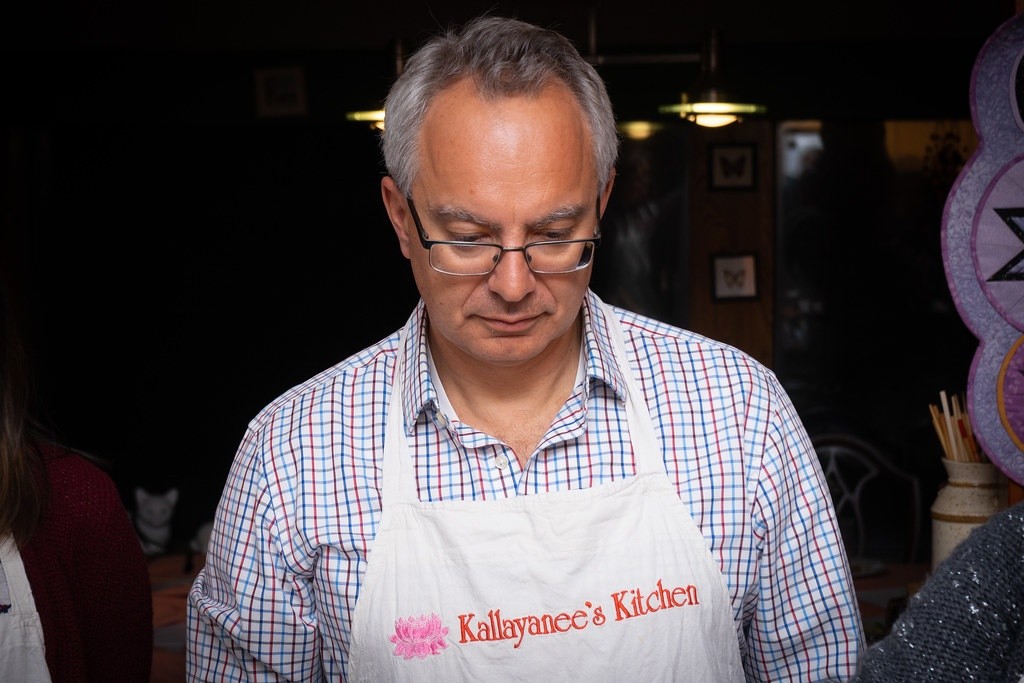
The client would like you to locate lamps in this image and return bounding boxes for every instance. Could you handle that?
[342,91,386,131]
[616,106,670,147]
[655,76,771,130]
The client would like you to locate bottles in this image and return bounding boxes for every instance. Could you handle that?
[929,458,1005,576]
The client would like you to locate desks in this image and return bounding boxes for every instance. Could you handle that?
[143,555,938,683]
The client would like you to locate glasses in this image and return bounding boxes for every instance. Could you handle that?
[405,194,602,276]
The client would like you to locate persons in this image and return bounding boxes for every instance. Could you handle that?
[0,341,155,683]
[185,18,866,683]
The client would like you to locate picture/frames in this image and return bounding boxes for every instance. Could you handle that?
[706,145,758,192]
[712,254,759,303]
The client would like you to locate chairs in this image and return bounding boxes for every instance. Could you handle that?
[14,416,156,683]
[814,432,923,562]
